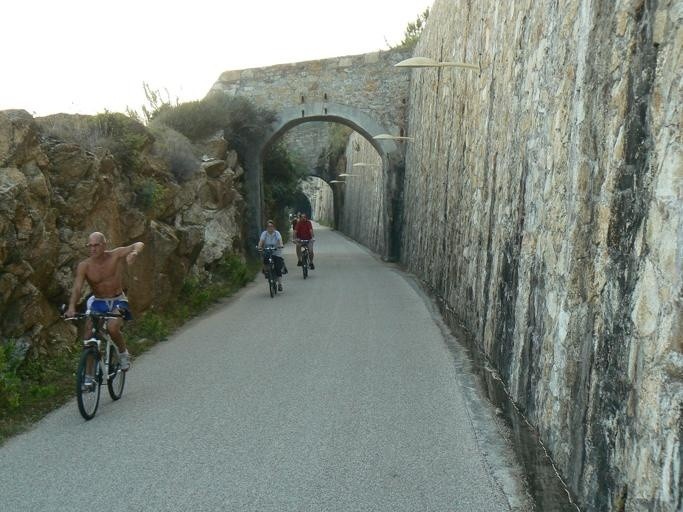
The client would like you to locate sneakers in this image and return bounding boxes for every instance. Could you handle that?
[120,349,131,372]
[278,283,282,291]
[309,263,314,269]
[297,260,302,266]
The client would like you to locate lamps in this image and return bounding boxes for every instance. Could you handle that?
[353,163,376,168]
[339,173,359,177]
[330,180,346,184]
[394,55,481,78]
[373,134,415,144]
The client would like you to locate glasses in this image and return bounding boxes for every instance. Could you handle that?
[87,243,102,247]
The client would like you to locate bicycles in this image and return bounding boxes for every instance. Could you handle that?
[61,304,129,420]
[261,244,283,296]
[295,238,313,278]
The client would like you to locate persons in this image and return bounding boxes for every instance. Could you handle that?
[64,232,145,390]
[292,214,315,270]
[258,220,284,291]
